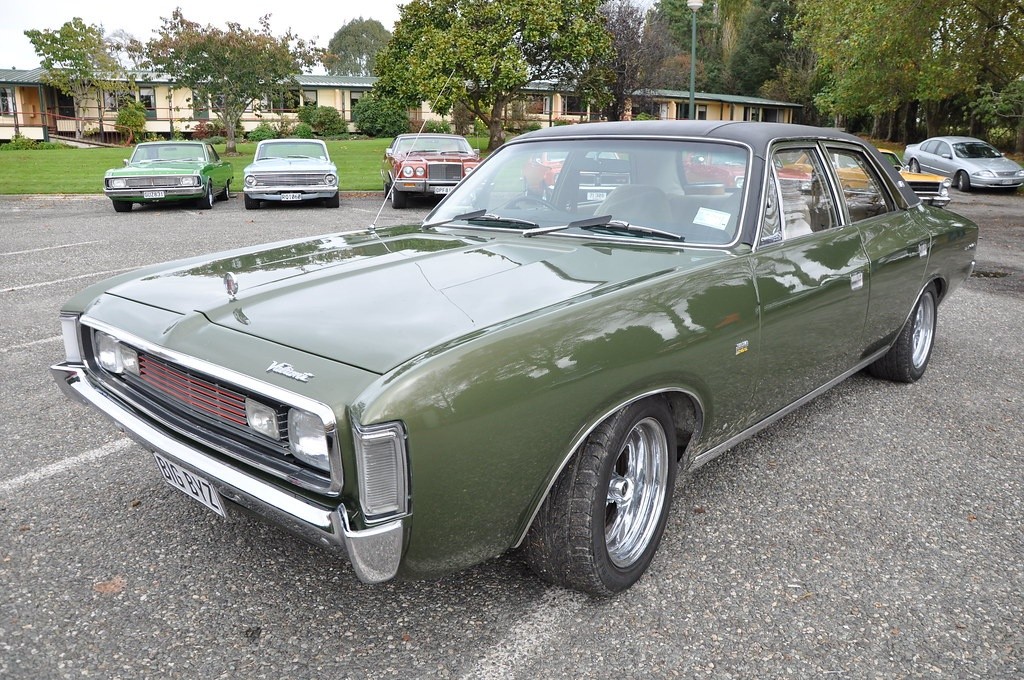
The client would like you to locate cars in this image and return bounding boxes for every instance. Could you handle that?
[102,141,233,212]
[379,132,489,211]
[683,146,813,200]
[904,134,1024,195]
[780,145,953,209]
[519,144,632,212]
[240,138,341,210]
[48,118,986,600]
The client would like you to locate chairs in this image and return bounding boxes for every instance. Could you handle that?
[592,184,673,233]
[714,192,813,242]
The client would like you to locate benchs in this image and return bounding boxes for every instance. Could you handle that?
[670,191,886,242]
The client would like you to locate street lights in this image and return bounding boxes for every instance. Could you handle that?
[685,1,705,119]
